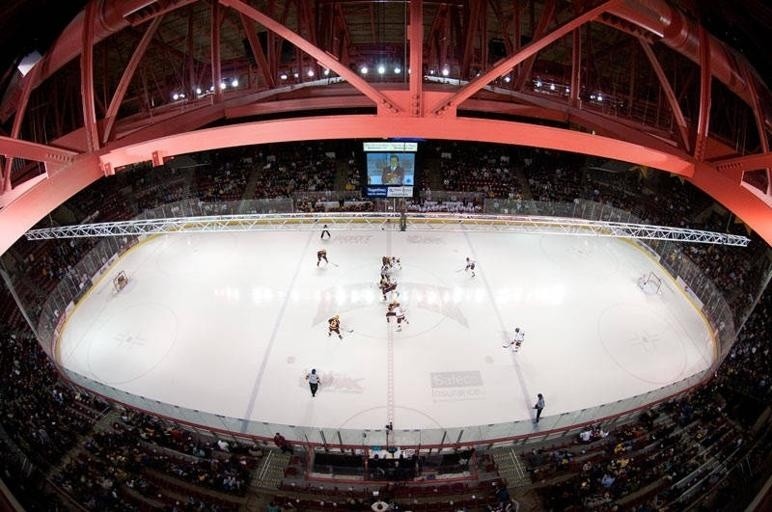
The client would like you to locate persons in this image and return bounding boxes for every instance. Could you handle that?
[0,139,772,512]
